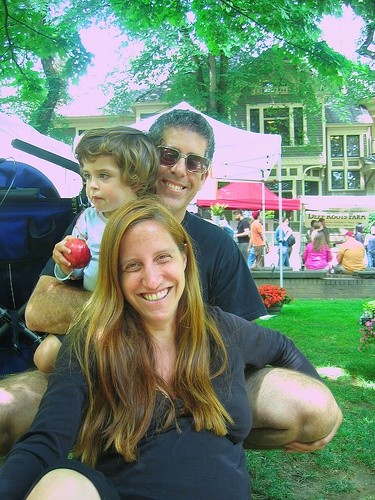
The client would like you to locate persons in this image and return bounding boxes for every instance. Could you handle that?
[0,110,375,500]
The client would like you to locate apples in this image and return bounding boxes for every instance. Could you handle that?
[62,235,91,268]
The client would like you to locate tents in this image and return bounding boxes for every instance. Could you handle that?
[0,102,375,288]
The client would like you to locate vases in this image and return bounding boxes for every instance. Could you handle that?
[268,301,284,313]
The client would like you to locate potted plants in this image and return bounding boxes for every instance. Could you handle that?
[207,203,228,224]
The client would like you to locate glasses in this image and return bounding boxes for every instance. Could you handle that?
[157,145,210,175]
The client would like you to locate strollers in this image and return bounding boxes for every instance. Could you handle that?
[0,139,93,378]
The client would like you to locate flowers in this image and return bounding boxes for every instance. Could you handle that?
[256,282,295,309]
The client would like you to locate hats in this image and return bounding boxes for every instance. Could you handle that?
[345,231,355,237]
[252,211,259,220]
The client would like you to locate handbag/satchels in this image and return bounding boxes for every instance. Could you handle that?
[282,234,296,247]
